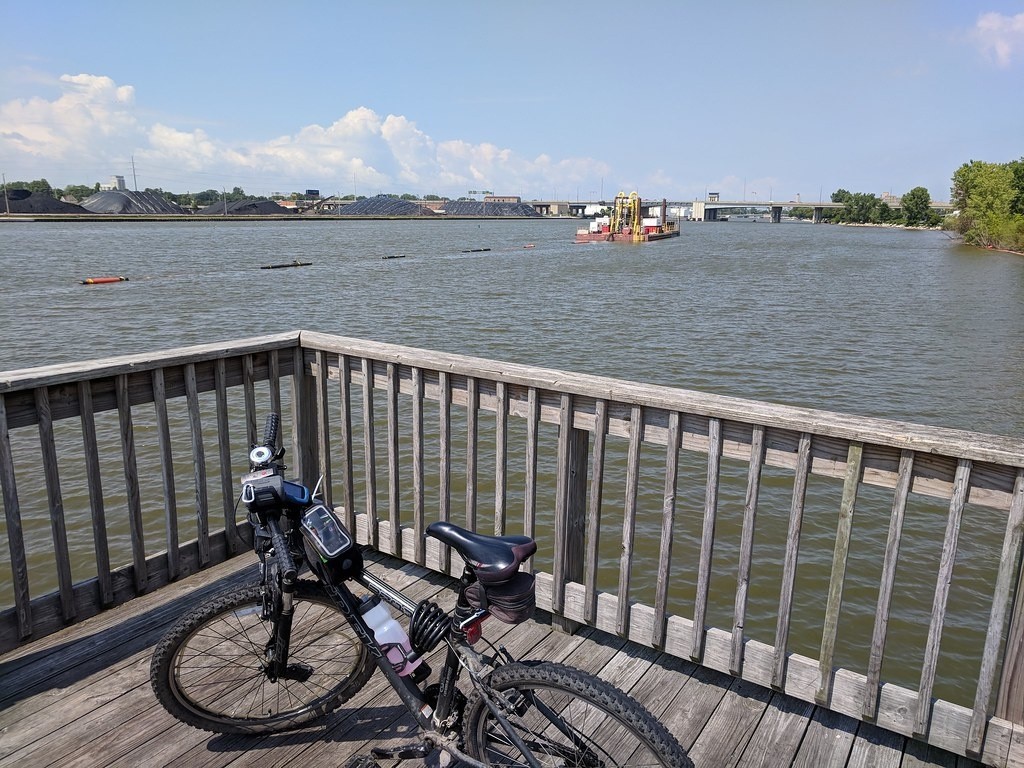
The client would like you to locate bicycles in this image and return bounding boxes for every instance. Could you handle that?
[148,410,700,768]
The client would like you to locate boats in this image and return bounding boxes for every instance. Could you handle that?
[573,190,682,241]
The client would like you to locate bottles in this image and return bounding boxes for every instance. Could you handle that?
[358,593,423,677]
